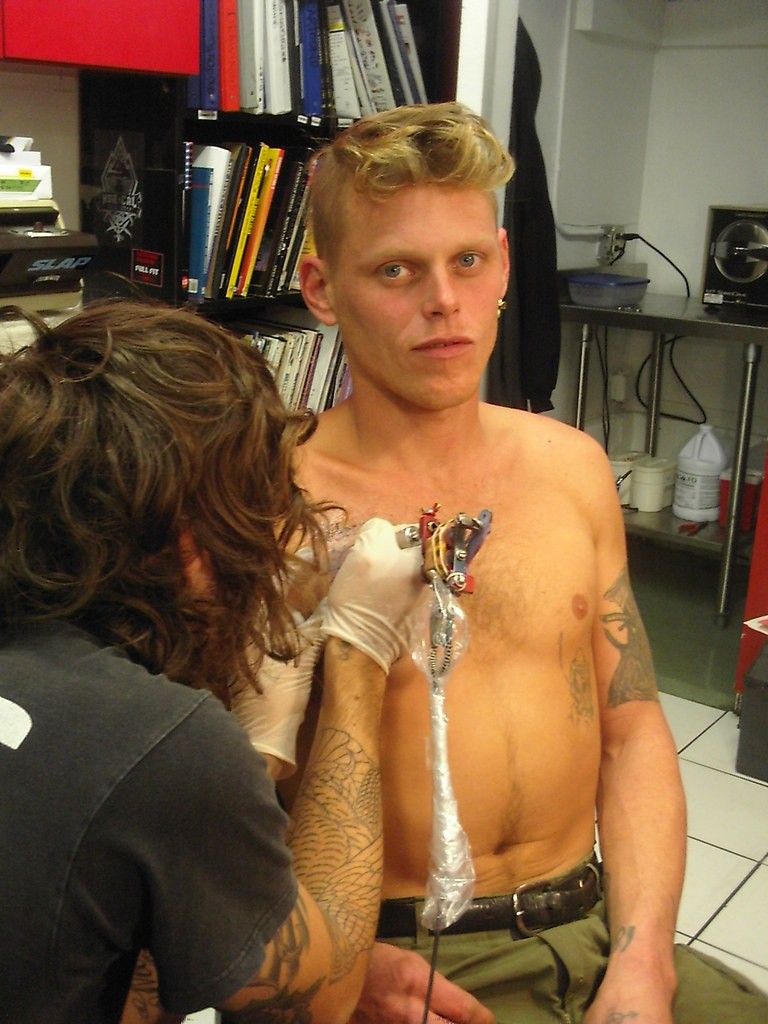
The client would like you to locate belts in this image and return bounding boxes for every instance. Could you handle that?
[373,854,608,938]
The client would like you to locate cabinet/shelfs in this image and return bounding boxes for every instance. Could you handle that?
[557,289,768,629]
[78,0,463,322]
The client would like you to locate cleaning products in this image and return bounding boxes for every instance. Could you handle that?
[673,418,727,521]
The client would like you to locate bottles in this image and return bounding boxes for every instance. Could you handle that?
[671,423,726,522]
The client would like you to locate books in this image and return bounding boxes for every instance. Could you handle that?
[200,1,430,121]
[218,303,353,415]
[176,139,326,301]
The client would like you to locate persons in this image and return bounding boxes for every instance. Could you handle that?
[0,298,433,1024]
[118,98,767,1023]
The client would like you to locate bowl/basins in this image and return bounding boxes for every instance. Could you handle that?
[568,272,652,307]
